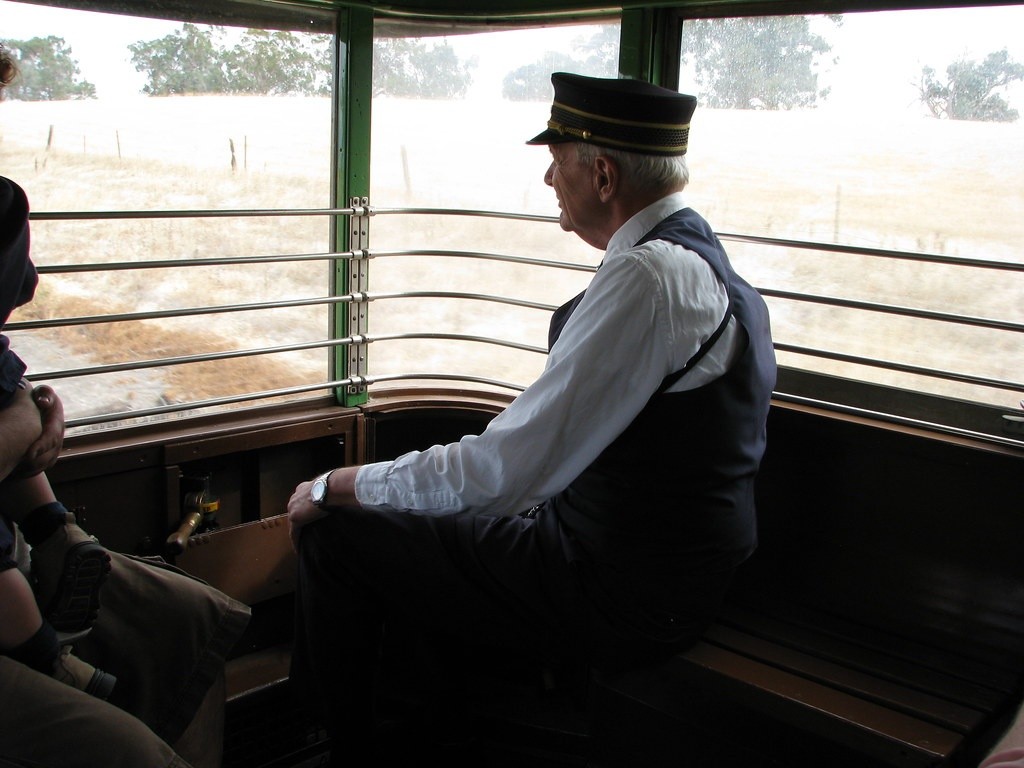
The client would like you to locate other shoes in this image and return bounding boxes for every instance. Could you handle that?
[29,512,111,633]
[47,645,117,701]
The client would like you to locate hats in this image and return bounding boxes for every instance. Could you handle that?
[526,73,698,156]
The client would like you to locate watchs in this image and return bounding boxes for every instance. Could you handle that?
[307,470,334,506]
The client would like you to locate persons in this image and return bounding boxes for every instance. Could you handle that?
[0,177,252,768]
[286,79,779,768]
